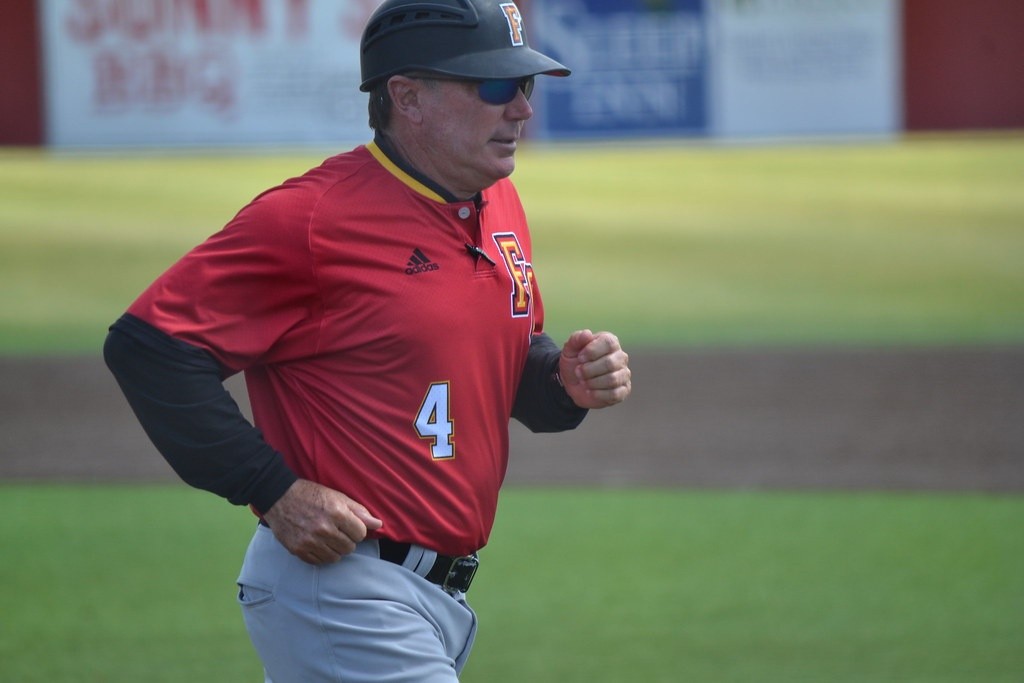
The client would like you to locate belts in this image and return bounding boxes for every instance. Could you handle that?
[379,537,481,594]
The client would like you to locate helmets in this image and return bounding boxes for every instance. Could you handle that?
[359,0,572,92]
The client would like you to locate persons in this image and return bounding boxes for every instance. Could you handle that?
[99,0,632,683]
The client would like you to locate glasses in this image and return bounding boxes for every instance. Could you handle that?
[406,75,536,105]
